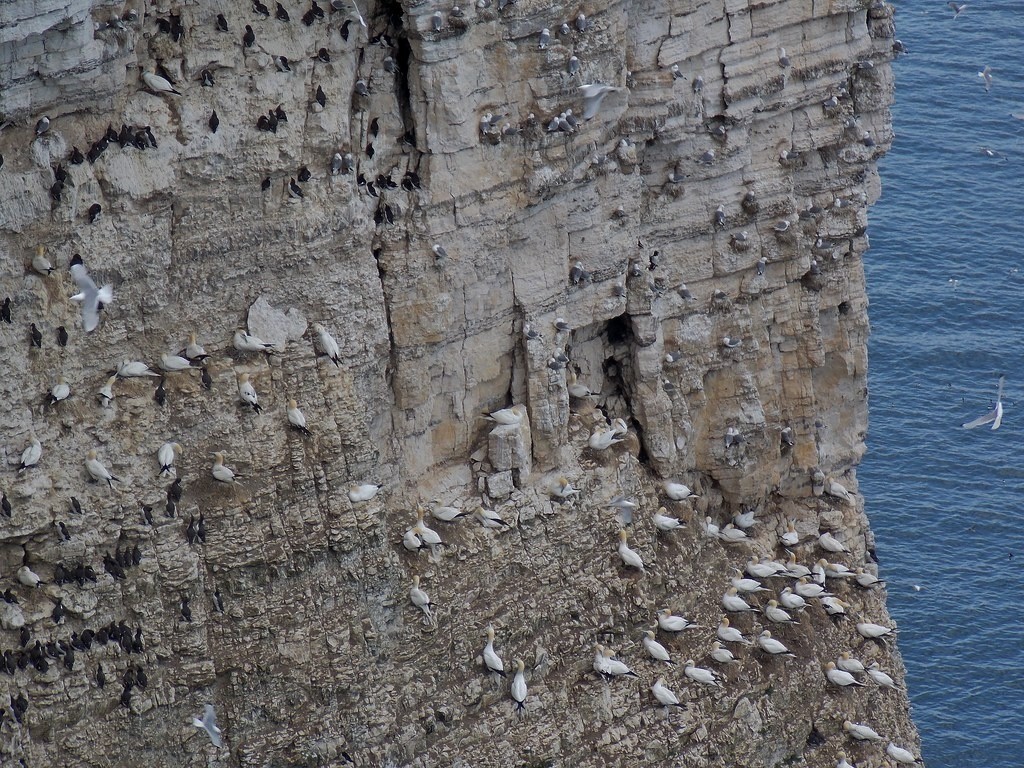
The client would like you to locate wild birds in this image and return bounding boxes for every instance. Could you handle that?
[0,0,1004,766]
